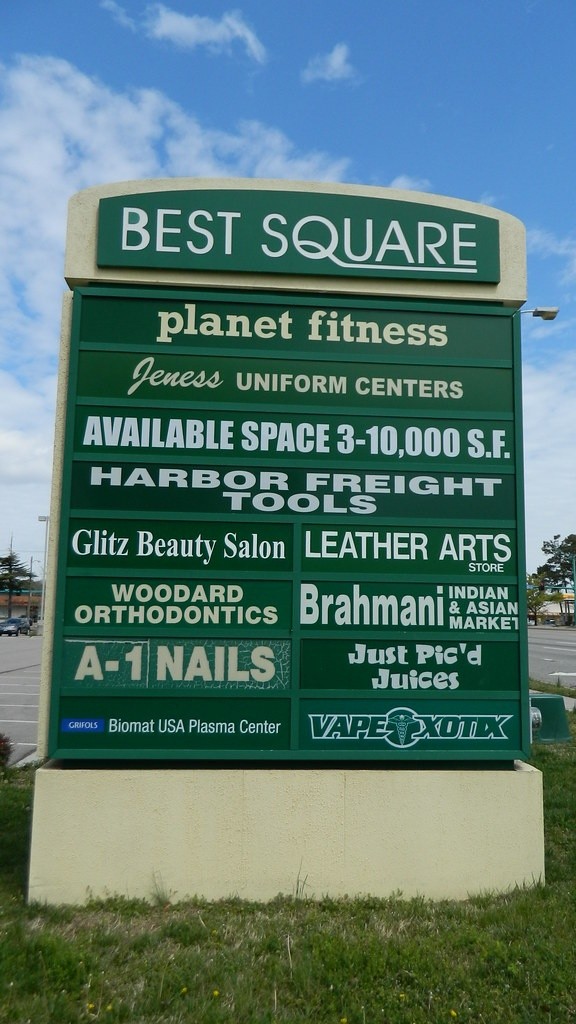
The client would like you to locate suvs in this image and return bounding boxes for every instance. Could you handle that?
[0,618,30,636]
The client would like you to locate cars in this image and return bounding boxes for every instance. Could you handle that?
[545,618,555,623]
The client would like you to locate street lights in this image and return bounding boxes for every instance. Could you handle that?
[27,556,41,618]
[38,515,50,620]
